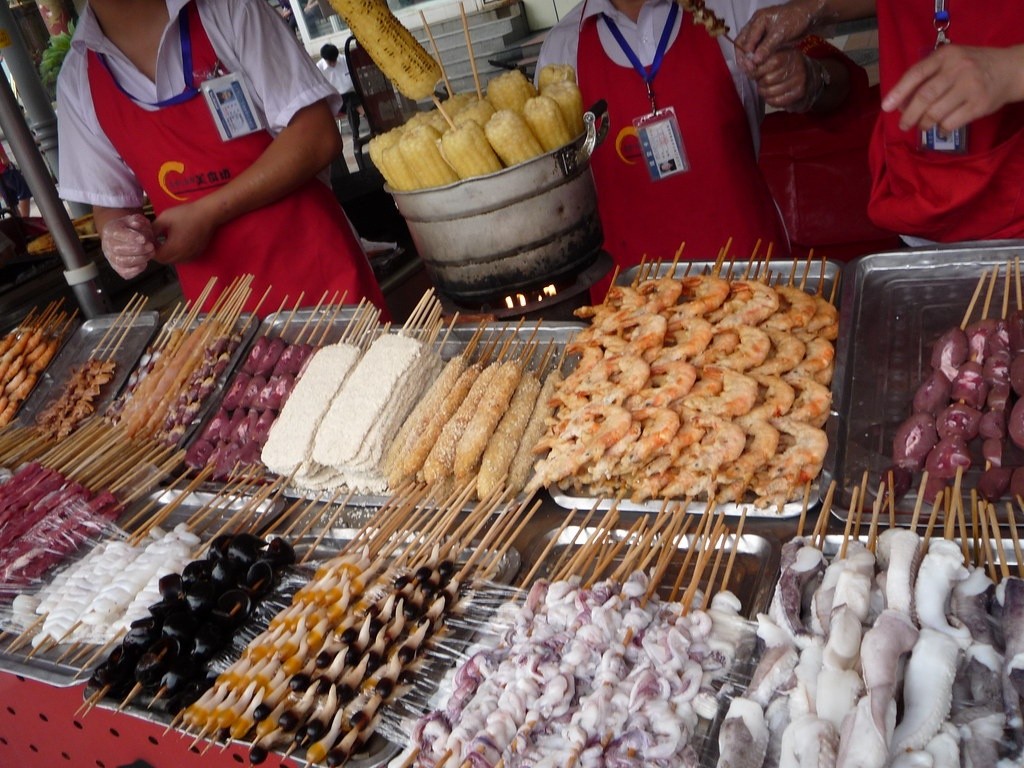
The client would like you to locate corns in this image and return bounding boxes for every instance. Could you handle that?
[327,0,443,101]
[367,63,583,189]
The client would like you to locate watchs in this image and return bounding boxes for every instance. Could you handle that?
[813,60,831,95]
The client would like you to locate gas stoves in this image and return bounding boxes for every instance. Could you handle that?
[437,251,610,323]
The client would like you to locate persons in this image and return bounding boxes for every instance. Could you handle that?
[217,91,233,102]
[55,0,391,323]
[533,0,851,275]
[937,134,952,143]
[0,143,33,218]
[733,0,1024,240]
[316,44,366,139]
[660,160,676,172]
[279,0,318,38]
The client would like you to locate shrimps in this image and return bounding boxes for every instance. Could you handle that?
[525,275,837,514]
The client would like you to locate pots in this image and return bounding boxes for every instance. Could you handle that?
[381,101,612,302]
